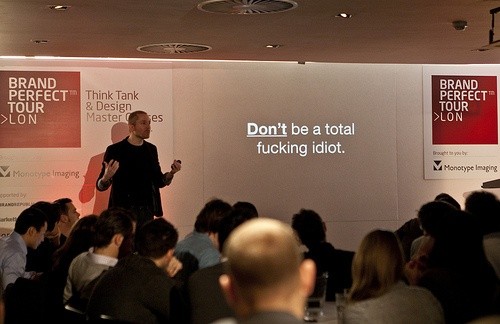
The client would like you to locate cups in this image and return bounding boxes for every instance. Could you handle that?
[336,293,349,324]
[304,274,328,321]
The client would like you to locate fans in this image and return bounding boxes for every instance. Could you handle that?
[479,6,500,51]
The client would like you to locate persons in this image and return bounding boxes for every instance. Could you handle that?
[96,110,181,231]
[0,190,500,324]
